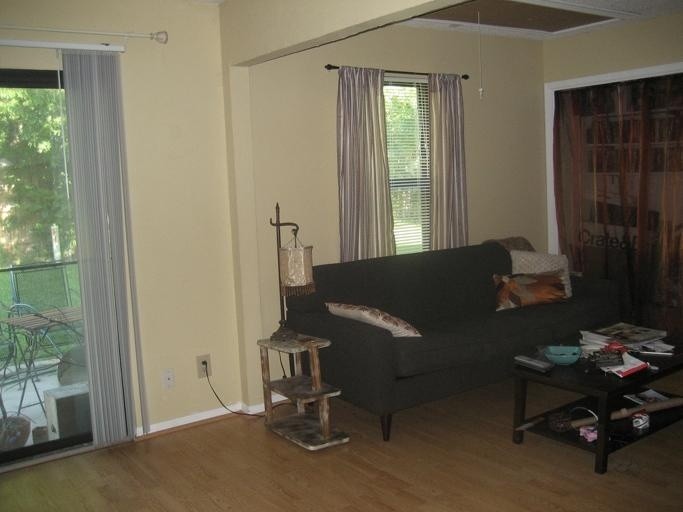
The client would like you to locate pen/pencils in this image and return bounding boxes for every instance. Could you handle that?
[640,351,674,355]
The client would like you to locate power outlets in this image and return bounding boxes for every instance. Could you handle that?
[195,354,214,380]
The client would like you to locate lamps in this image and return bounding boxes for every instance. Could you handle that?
[268,201,318,344]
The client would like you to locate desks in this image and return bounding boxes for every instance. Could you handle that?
[1,294,88,421]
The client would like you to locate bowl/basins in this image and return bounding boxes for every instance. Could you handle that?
[544,346,580,365]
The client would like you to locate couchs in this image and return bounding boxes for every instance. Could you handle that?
[276,236,623,441]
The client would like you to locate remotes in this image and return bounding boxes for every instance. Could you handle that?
[515,354,552,372]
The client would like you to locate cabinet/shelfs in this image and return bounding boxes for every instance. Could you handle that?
[256,335,349,450]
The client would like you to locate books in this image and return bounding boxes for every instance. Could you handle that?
[577,321,675,378]
[621,387,671,406]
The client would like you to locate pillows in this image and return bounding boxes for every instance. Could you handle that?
[491,272,567,311]
[323,295,421,337]
[511,250,571,298]
